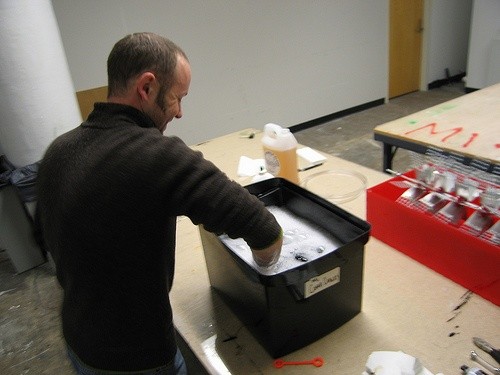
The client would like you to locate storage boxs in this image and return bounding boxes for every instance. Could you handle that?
[200,178,371,358]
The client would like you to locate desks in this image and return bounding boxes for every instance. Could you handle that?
[373,83,500,176]
[169,128,500,375]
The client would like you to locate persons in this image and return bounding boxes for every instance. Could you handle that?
[36,32,284,375]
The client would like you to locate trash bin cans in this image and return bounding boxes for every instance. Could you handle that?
[0,151,54,275]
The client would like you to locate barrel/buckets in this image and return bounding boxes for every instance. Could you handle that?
[263,123,298,186]
